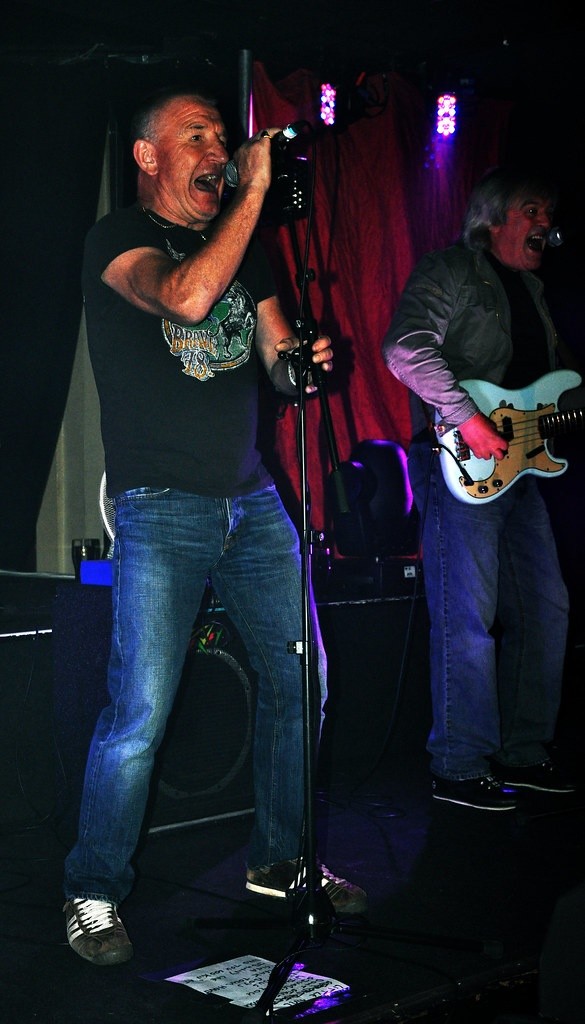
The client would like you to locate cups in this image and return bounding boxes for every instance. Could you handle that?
[72,538,100,584]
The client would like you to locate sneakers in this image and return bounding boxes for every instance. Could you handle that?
[63,898,133,966]
[432,773,520,810]
[503,759,576,792]
[246,857,367,913]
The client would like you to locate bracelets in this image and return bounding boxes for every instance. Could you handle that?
[287,362,296,386]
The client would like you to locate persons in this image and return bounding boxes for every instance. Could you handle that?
[383,176,581,811]
[62,83,334,965]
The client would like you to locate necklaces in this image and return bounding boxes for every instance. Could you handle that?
[141,205,207,242]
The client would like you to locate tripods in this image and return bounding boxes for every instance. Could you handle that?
[193,168,508,1024]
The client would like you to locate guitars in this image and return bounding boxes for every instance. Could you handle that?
[435,369,585,506]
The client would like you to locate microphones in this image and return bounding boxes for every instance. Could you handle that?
[547,226,564,246]
[223,122,306,187]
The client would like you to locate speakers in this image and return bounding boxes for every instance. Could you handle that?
[53,581,257,839]
[313,592,435,789]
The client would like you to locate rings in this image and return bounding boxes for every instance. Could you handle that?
[262,128,272,139]
